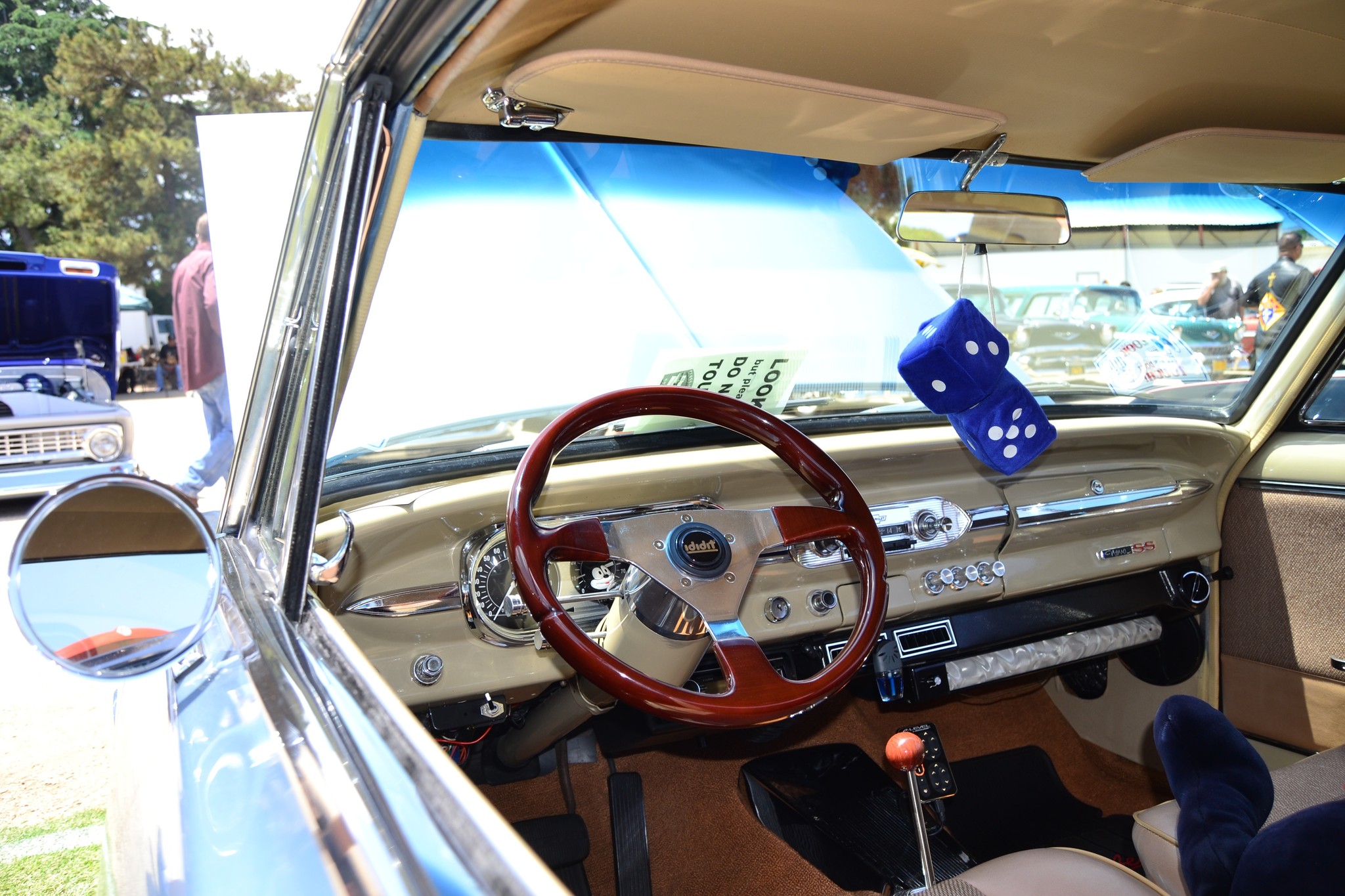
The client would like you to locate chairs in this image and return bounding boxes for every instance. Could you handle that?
[1128,743,1345,896]
[889,826,1171,896]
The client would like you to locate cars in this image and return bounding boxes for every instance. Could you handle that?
[4,0,1345,896]
[937,267,1345,422]
[0,247,139,502]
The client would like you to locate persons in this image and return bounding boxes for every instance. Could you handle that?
[1242,233,1315,371]
[171,212,235,510]
[1198,260,1244,322]
[156,334,183,392]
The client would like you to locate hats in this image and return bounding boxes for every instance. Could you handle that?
[1211,259,1227,274]
